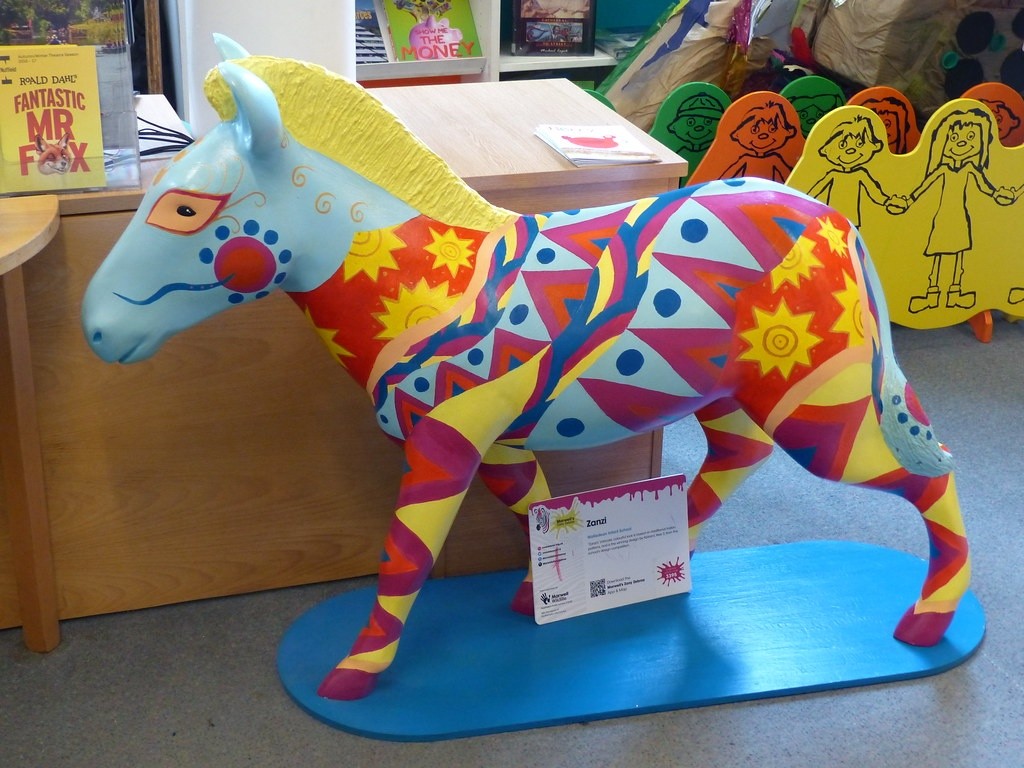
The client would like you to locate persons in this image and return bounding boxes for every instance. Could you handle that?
[525,23,582,41]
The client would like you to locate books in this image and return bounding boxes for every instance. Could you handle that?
[596,26,652,59]
[501,0,597,56]
[355,0,482,65]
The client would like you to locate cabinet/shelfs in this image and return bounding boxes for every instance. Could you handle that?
[0,0,687,629]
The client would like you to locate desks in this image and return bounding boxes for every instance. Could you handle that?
[0,195,62,653]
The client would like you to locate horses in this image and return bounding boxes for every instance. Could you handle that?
[74,28,975,704]
